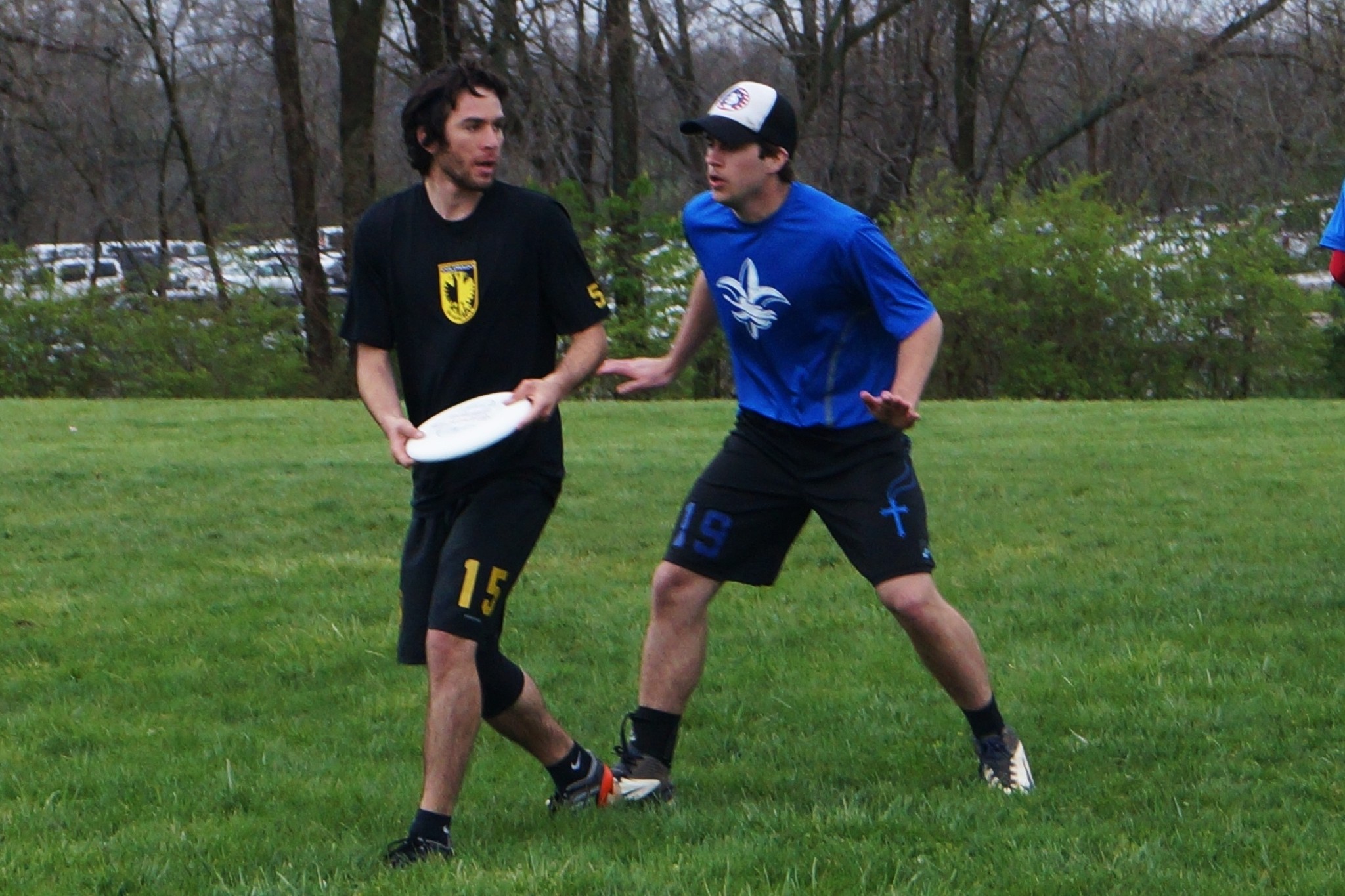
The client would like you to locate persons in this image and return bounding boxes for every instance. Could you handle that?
[1320,181,1345,288]
[594,81,1034,804]
[340,67,618,868]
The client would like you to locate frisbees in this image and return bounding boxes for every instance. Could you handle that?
[405,391,533,462]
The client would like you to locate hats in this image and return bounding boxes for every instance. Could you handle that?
[679,80,800,155]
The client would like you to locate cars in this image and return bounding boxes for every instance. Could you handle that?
[0,225,348,309]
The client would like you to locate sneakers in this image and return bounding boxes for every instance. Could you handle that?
[602,743,677,809]
[384,834,457,873]
[542,748,622,826]
[976,727,1040,799]
